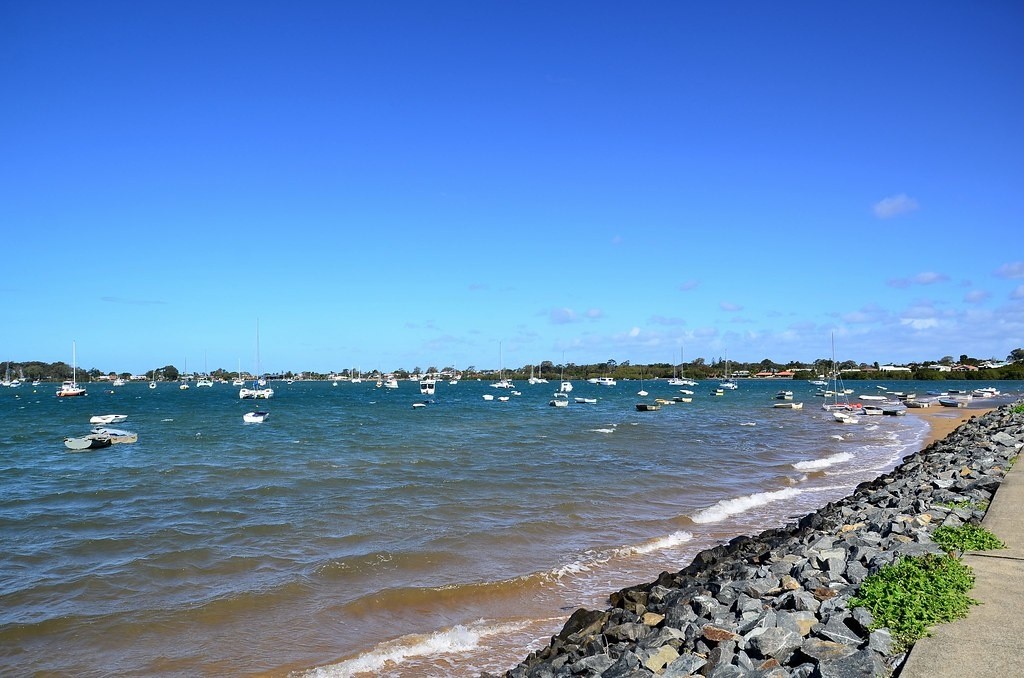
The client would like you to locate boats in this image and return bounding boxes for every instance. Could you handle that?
[384,374,399,388]
[240,319,275,400]
[938,399,966,408]
[528,361,549,385]
[927,389,966,395]
[635,389,695,411]
[971,386,1001,398]
[63,415,138,450]
[877,384,931,408]
[807,332,907,424]
[243,411,269,424]
[548,351,573,407]
[709,349,738,396]
[113,376,126,386]
[773,403,804,409]
[491,340,515,388]
[586,374,616,386]
[420,378,435,394]
[858,394,888,400]
[776,390,793,400]
[196,349,213,388]
[574,398,597,405]
[0,360,21,387]
[56,340,86,396]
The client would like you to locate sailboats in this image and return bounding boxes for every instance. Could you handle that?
[667,346,698,387]
[637,371,648,397]
